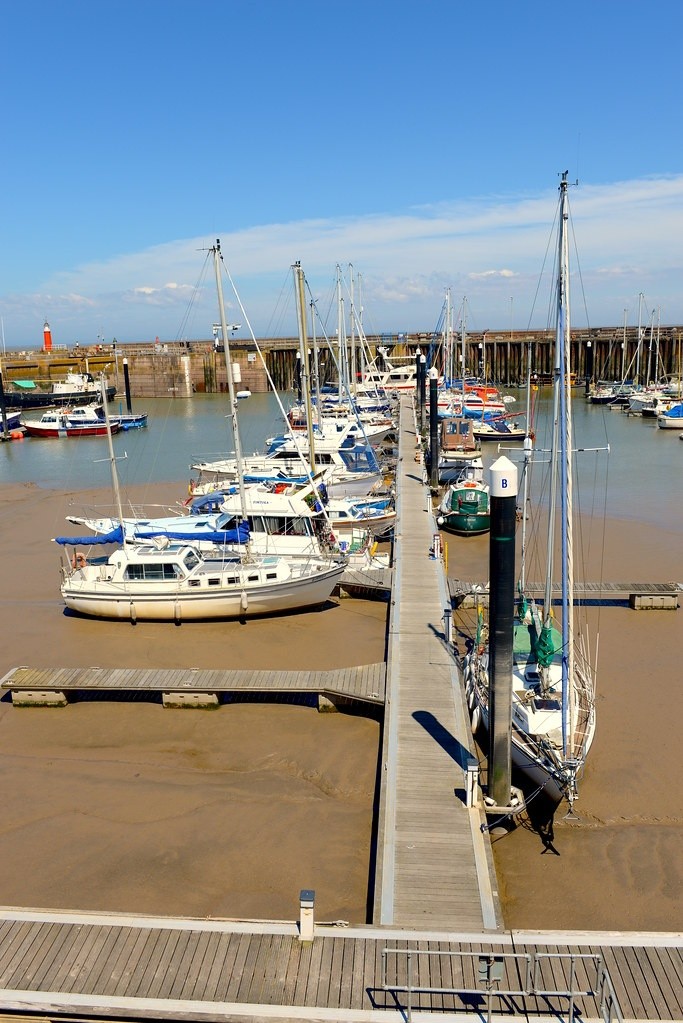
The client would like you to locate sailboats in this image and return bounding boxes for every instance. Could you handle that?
[52,236,683,624]
[464,170,599,817]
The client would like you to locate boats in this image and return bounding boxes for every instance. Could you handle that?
[50,363,117,407]
[20,414,120,438]
[40,400,149,430]
[0,411,27,442]
[0,357,118,410]
